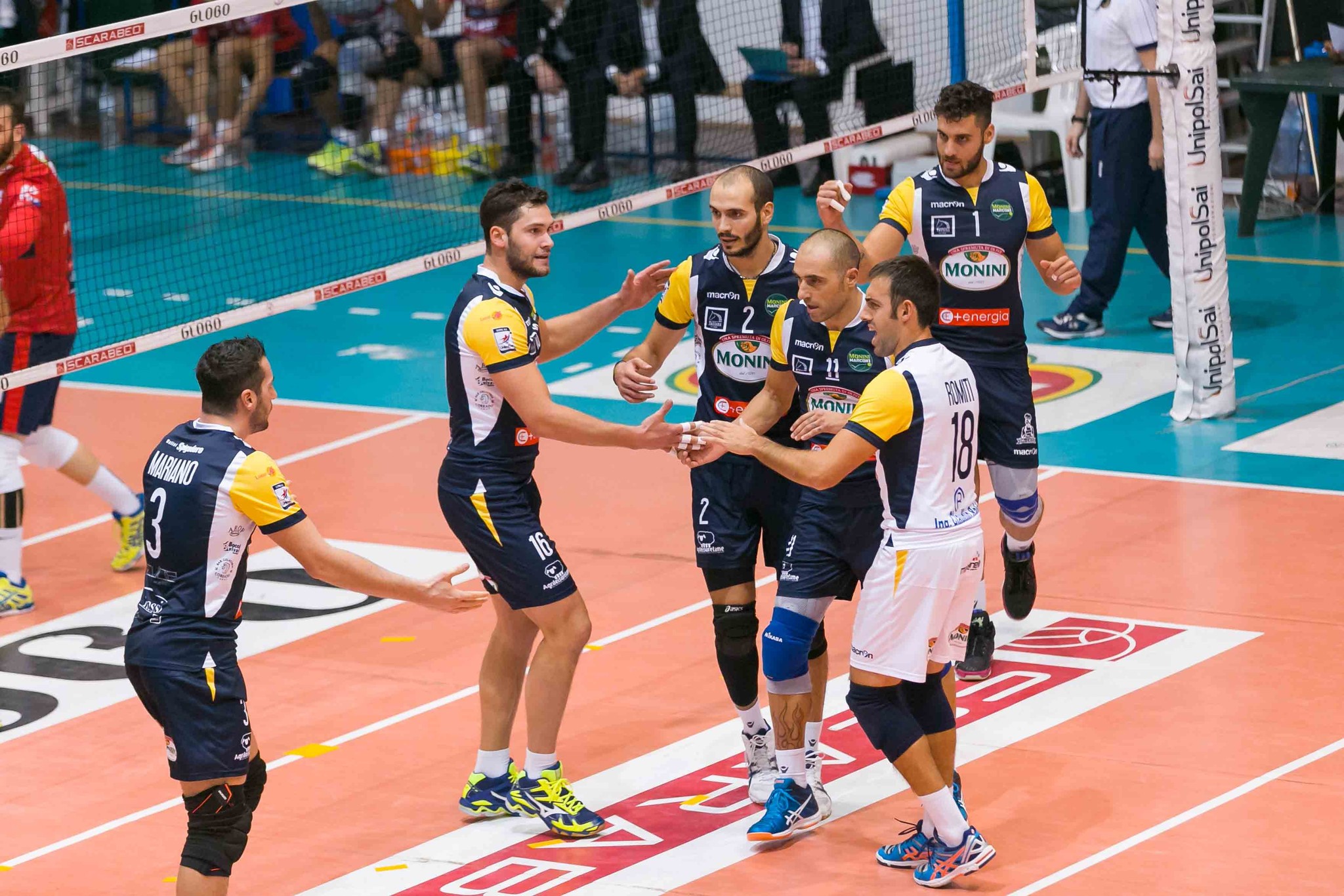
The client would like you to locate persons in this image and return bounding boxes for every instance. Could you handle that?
[568,0,725,193]
[436,182,711,838]
[0,0,516,179]
[0,86,146,617]
[611,165,834,821]
[674,229,969,842]
[814,81,1083,680]
[124,335,490,896]
[492,0,605,186]
[743,0,888,197]
[1324,40,1344,63]
[1035,0,1173,340]
[698,256,998,889]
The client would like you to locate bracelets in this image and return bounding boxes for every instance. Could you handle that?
[611,360,625,387]
[1070,114,1087,124]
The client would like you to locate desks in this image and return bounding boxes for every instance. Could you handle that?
[1231,54,1344,235]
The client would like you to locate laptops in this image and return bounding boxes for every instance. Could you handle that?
[736,46,798,75]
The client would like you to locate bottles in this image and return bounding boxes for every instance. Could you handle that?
[98,83,118,147]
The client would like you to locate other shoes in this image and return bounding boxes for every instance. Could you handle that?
[306,140,349,169]
[461,149,490,177]
[492,156,533,179]
[766,165,800,187]
[571,169,609,191]
[668,165,697,182]
[320,148,364,175]
[187,144,244,172]
[161,136,215,165]
[803,174,834,196]
[350,143,388,177]
[553,160,583,183]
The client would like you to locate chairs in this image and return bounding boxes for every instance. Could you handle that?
[43,0,895,192]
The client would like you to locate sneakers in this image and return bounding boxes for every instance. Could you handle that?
[746,777,820,841]
[505,760,605,836]
[875,818,936,866]
[111,492,145,572]
[0,571,35,617]
[1149,307,1173,328]
[741,719,780,804]
[804,750,832,820]
[953,769,969,825]
[458,757,539,818]
[1000,530,1037,620]
[913,825,996,888]
[954,610,995,680]
[1036,310,1105,339]
